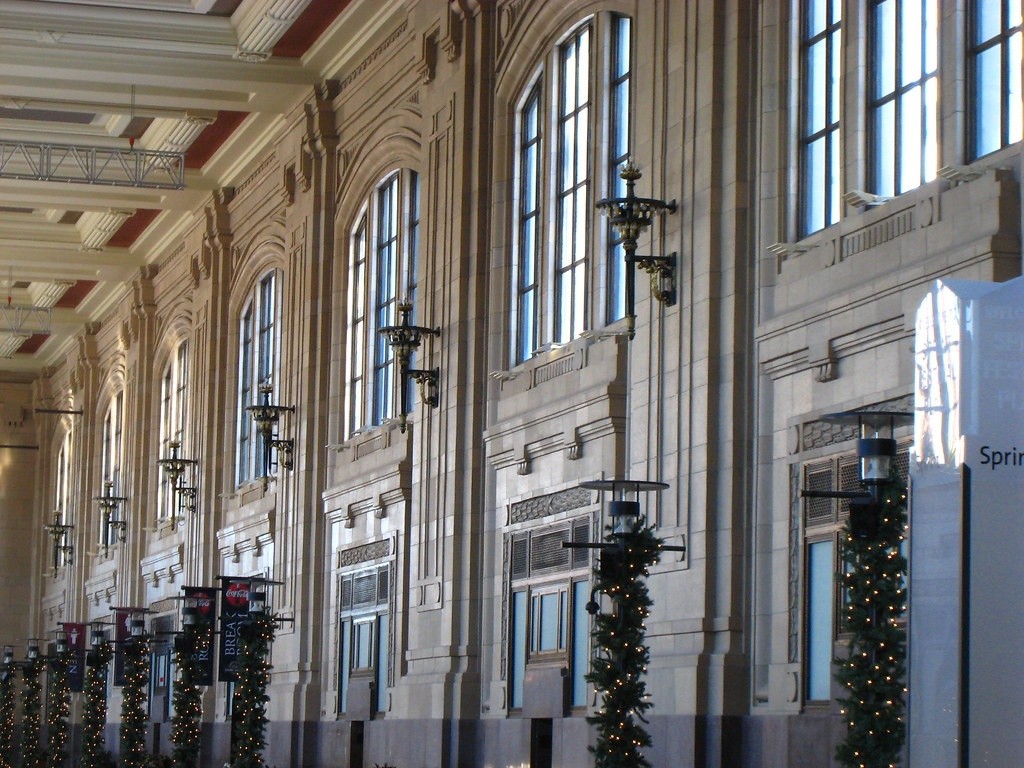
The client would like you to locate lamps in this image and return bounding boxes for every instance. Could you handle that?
[43,510,74,579]
[167,596,205,623]
[799,411,915,504]
[27,638,42,657]
[378,299,440,435]
[244,379,294,491]
[116,610,184,635]
[52,631,69,652]
[559,480,686,554]
[154,433,198,533]
[81,621,120,644]
[593,162,677,340]
[3,645,17,663]
[93,480,128,558]
[217,576,294,625]
